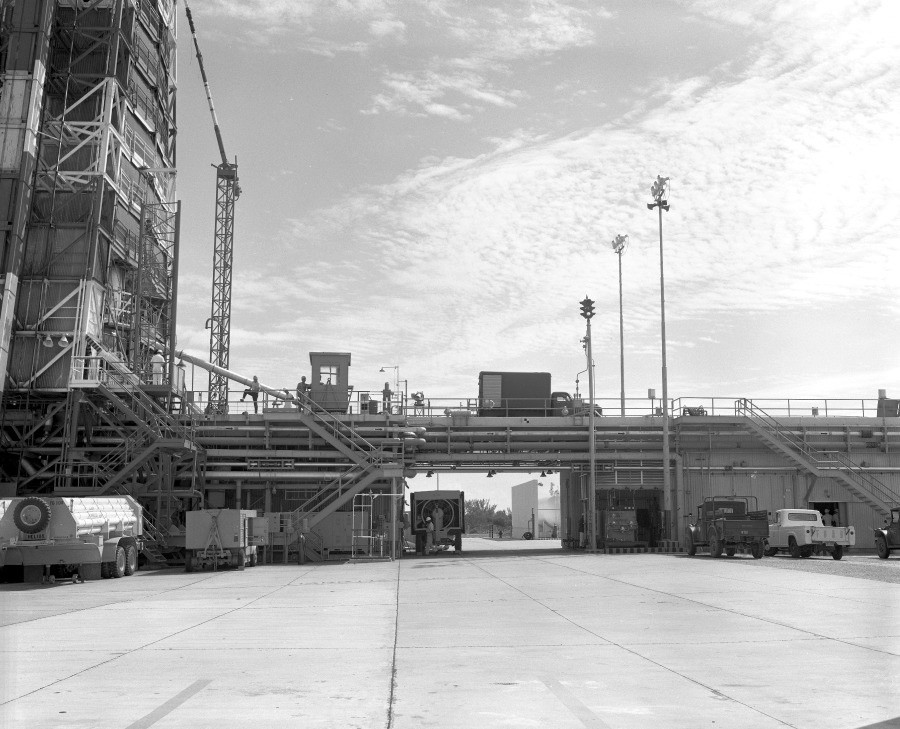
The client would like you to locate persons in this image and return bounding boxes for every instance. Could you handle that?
[425,517,435,555]
[283,388,294,407]
[296,375,311,410]
[822,509,839,527]
[240,375,259,414]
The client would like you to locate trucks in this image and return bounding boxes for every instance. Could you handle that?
[478,371,602,416]
[873,506,900,558]
[410,490,465,553]
[684,495,770,559]
[764,509,856,560]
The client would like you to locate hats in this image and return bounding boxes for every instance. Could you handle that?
[253,375,258,379]
[834,509,838,512]
[825,509,829,512]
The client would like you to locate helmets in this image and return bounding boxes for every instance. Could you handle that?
[426,516,431,521]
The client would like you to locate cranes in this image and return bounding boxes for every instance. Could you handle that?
[185,1,242,416]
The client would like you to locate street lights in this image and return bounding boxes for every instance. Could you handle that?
[611,233,629,417]
[648,175,672,541]
[178,360,186,411]
[579,296,596,551]
[426,472,439,490]
[380,367,407,415]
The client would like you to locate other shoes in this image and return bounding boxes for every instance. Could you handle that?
[240,399,244,402]
